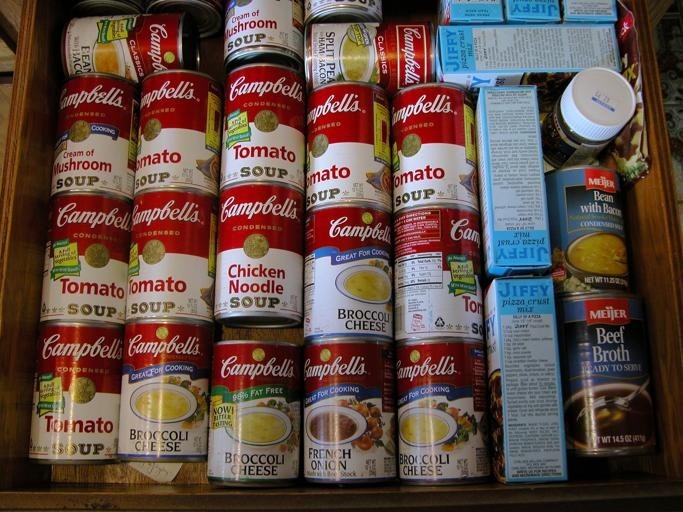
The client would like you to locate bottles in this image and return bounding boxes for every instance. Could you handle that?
[540,67,639,169]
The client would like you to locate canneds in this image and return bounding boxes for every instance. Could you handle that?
[560,296,658,456]
[219,62,305,189]
[213,181,302,328]
[301,203,392,339]
[119,316,210,465]
[393,206,485,341]
[307,0,384,23]
[392,82,478,206]
[549,165,634,294]
[49,71,132,200]
[310,81,393,211]
[127,187,211,324]
[76,2,140,13]
[398,339,488,485]
[38,191,125,327]
[208,340,300,488]
[138,71,216,195]
[27,323,118,467]
[62,13,201,71]
[306,339,396,484]
[146,0,220,41]
[305,22,432,86]
[222,0,304,64]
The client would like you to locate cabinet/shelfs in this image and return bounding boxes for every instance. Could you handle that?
[1,1,683,512]
